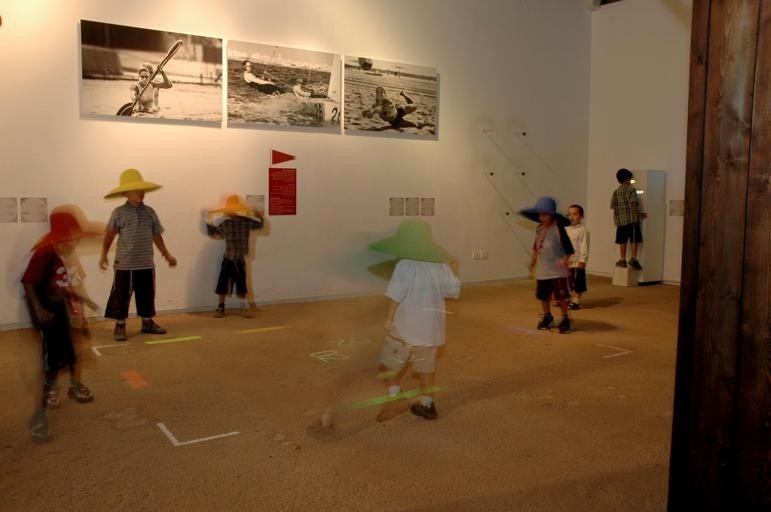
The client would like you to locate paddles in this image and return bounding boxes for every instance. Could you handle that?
[117,40,182,116]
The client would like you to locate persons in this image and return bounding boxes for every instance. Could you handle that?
[129,67,172,112]
[98,168,177,341]
[368,218,460,419]
[525,196,574,332]
[207,195,263,316]
[241,59,282,95]
[560,204,589,309]
[293,78,310,98]
[136,63,159,109]
[17,208,97,404]
[362,86,418,128]
[610,167,647,271]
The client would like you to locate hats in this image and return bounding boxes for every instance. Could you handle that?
[367,220,453,263]
[208,194,249,213]
[31,214,96,251]
[521,197,571,226]
[105,169,160,198]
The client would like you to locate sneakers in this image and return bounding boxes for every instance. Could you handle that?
[568,303,582,310]
[115,322,126,340]
[628,258,641,270]
[538,316,553,329]
[142,323,166,333]
[559,318,570,330]
[214,307,224,317]
[411,404,437,419]
[68,385,93,402]
[43,384,65,409]
[376,398,409,421]
[616,260,625,267]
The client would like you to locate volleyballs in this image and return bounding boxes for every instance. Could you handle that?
[359,58,373,70]
